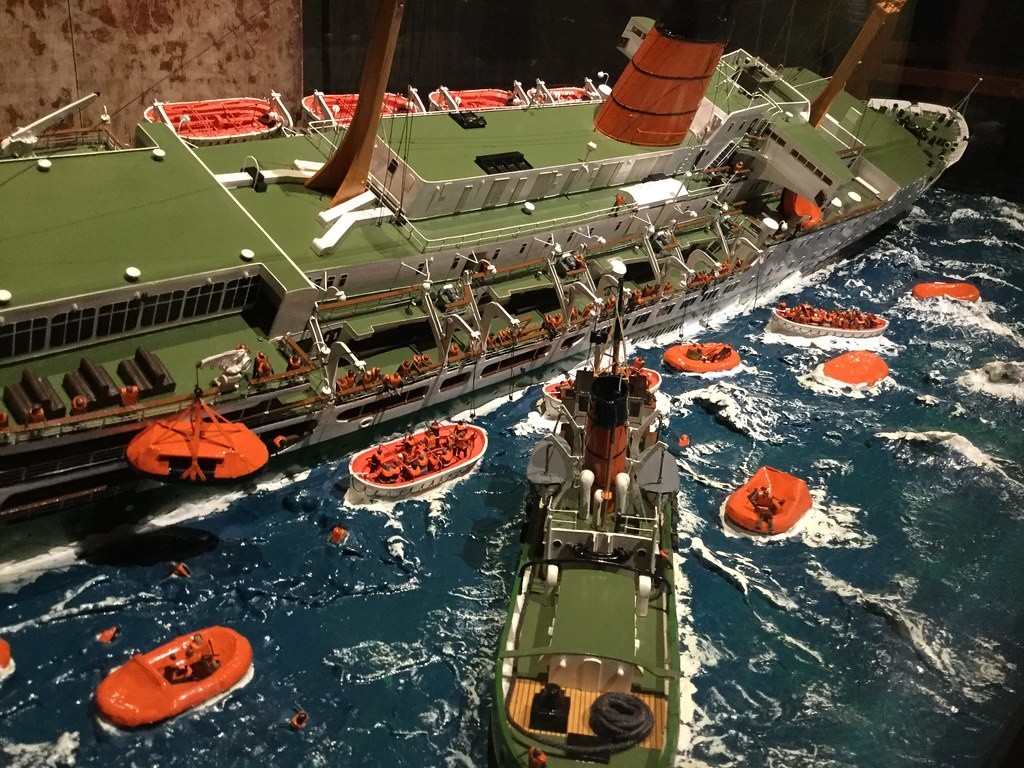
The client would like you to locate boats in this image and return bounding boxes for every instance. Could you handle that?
[300,94,421,124]
[811,350,889,390]
[663,343,741,374]
[492,278,684,767]
[0,14,970,583]
[770,307,890,339]
[724,465,813,535]
[92,625,254,729]
[122,427,271,479]
[349,422,489,498]
[913,282,980,304]
[526,86,593,106]
[143,97,285,147]
[427,88,524,112]
[541,366,662,413]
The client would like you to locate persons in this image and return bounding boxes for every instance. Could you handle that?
[168,634,218,681]
[686,342,732,363]
[370,419,470,482]
[734,161,744,170]
[98,625,122,643]
[332,523,349,544]
[290,711,309,728]
[0,383,140,429]
[555,379,574,398]
[539,281,674,328]
[336,327,519,393]
[609,195,625,215]
[529,746,547,768]
[777,302,882,330]
[274,434,290,450]
[618,356,653,391]
[749,485,781,534]
[235,341,304,391]
[693,258,745,284]
